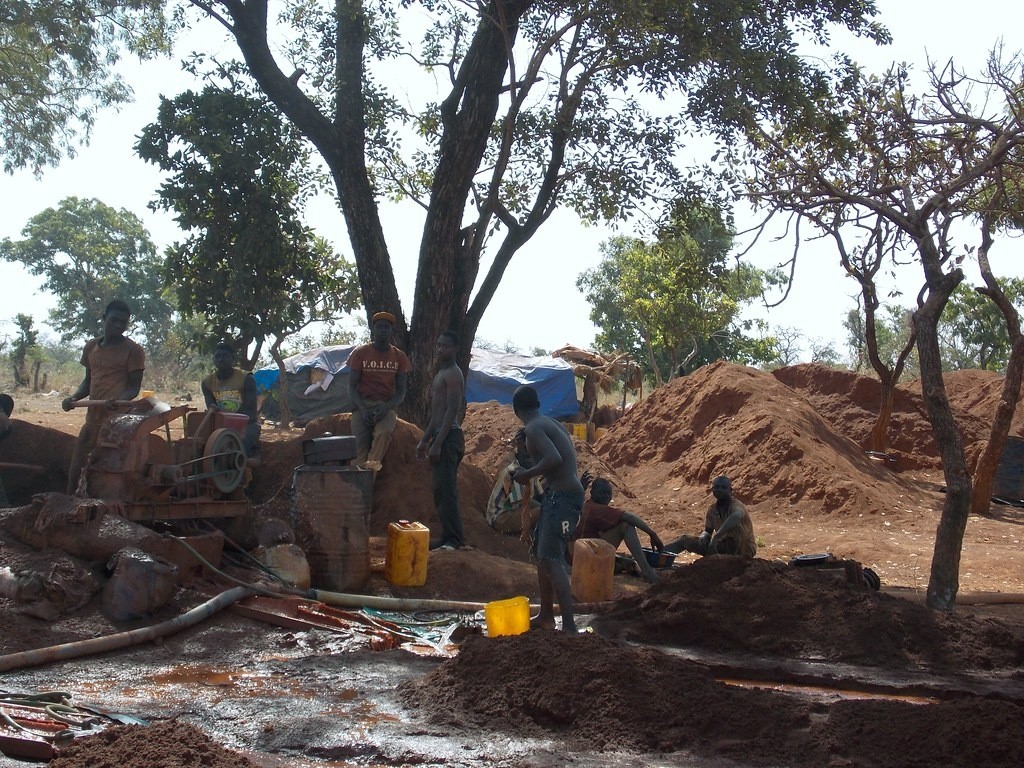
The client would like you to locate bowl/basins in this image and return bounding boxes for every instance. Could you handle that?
[638,547,679,569]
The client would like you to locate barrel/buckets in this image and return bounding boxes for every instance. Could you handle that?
[994,435,1024,501]
[483,595,531,639]
[292,465,374,594]
[573,423,587,441]
[142,391,154,399]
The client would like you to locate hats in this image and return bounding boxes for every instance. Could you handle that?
[372,312,396,325]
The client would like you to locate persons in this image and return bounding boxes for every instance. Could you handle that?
[513,386,585,634]
[0,394,14,440]
[63,300,144,495]
[415,332,465,550]
[665,475,756,557]
[567,471,665,580]
[202,344,257,454]
[488,445,542,533]
[345,312,413,472]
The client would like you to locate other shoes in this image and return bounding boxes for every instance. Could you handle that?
[430,542,454,553]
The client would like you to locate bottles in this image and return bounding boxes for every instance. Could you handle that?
[383,518,429,588]
[570,537,615,603]
